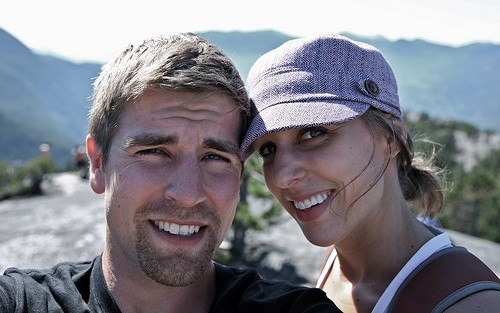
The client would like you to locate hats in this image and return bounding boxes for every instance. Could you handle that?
[238,33,402,161]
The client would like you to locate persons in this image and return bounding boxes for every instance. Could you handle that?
[0,32,342,312]
[67,141,93,181]
[240,34,500,313]
